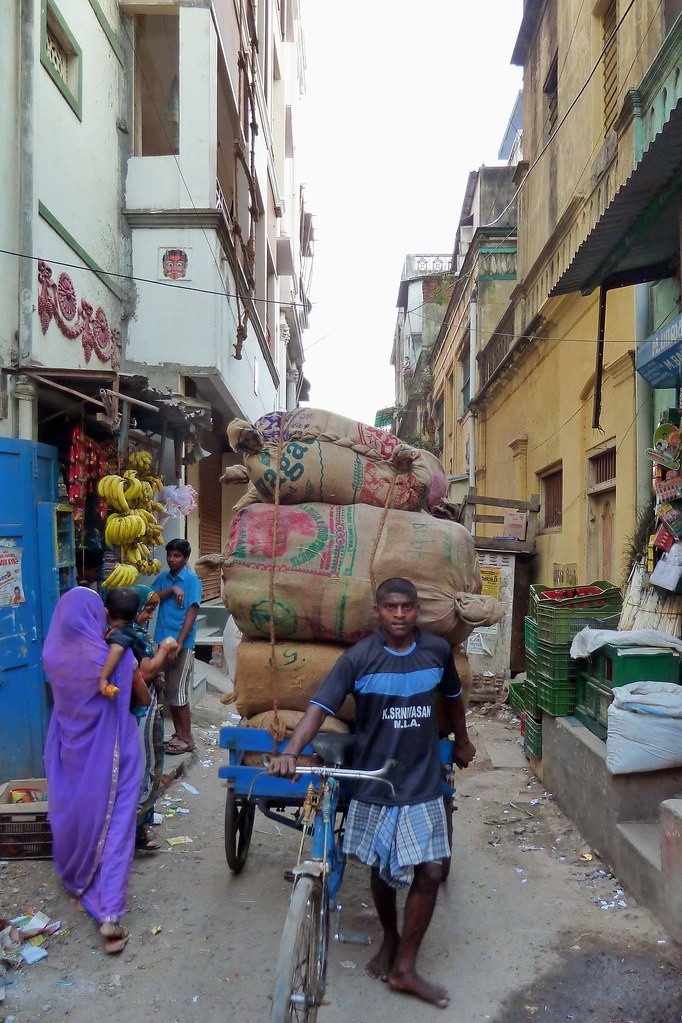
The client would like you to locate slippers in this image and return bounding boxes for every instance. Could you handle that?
[106,925,130,953]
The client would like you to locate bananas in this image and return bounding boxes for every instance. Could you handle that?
[98,448,167,590]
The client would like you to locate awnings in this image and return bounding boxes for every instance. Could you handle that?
[547,96,682,298]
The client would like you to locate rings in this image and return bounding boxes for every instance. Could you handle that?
[179,597,181,599]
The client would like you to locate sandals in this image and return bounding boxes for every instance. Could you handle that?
[163,732,197,754]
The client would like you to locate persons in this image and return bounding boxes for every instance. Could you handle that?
[42,587,152,954]
[129,583,179,851]
[267,577,477,1007]
[99,588,146,727]
[150,538,202,755]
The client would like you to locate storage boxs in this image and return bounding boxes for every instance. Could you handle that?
[509,581,623,763]
[0,778,55,860]
[502,511,527,542]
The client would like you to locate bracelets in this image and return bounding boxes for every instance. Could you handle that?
[161,640,170,651]
[284,752,298,760]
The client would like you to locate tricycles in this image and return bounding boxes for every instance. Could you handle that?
[218,681,476,1023]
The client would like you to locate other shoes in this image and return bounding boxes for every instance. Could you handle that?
[135,835,162,850]
